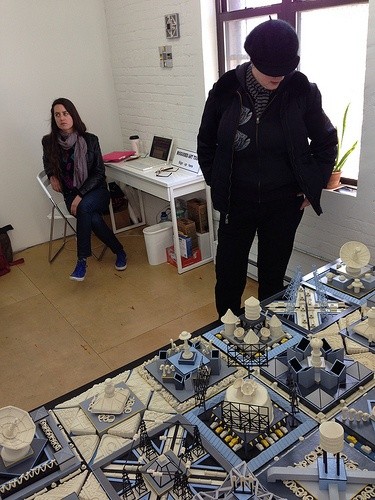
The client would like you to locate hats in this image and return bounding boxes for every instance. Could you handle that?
[244,15,301,77]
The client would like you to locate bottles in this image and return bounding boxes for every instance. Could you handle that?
[159,211,172,223]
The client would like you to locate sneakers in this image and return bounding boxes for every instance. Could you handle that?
[69,260,87,281]
[115,250,127,270]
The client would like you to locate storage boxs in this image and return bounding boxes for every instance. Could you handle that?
[102,208,131,232]
[166,198,212,268]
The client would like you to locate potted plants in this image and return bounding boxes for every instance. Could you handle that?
[326,103,358,189]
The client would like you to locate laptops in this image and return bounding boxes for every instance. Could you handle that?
[125,136,173,170]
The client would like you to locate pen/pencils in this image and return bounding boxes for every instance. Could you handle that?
[124,156,140,162]
[155,167,174,173]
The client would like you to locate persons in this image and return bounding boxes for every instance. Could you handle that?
[197,19,338,321]
[42,98,128,282]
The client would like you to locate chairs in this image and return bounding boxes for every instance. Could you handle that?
[36,170,106,264]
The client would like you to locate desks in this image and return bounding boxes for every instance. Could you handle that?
[103,156,215,275]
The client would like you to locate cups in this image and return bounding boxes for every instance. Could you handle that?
[129,135,140,157]
